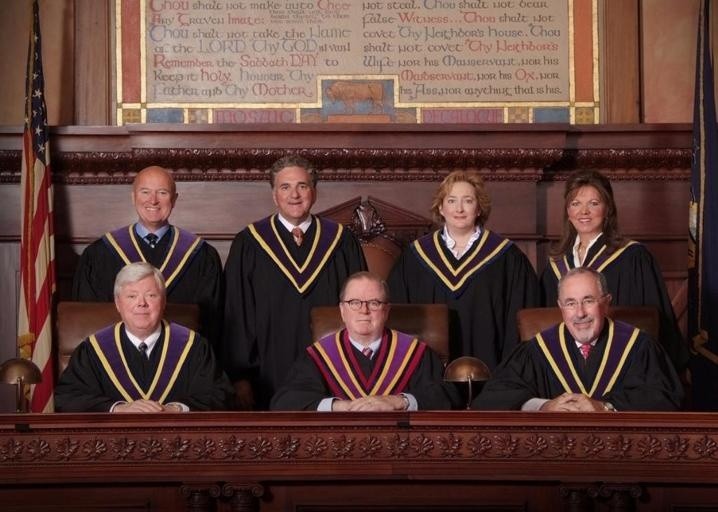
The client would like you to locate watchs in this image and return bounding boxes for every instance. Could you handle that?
[603,401,615,412]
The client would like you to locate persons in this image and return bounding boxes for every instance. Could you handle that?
[56,261,228,415]
[269,271,453,414]
[385,171,544,408]
[75,165,224,343]
[469,268,685,413]
[226,156,369,410]
[540,172,681,361]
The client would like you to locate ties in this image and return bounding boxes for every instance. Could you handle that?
[138,342,149,355]
[145,233,159,257]
[291,227,303,245]
[581,342,594,358]
[362,348,372,359]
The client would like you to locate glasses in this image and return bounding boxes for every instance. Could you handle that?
[343,298,389,311]
[557,299,598,308]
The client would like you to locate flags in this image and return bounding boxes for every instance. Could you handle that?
[13,4,57,415]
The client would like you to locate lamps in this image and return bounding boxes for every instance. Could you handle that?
[441,356,493,411]
[0,357,42,433]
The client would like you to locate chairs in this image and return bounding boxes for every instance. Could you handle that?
[517,305,660,342]
[310,303,450,372]
[55,301,200,382]
